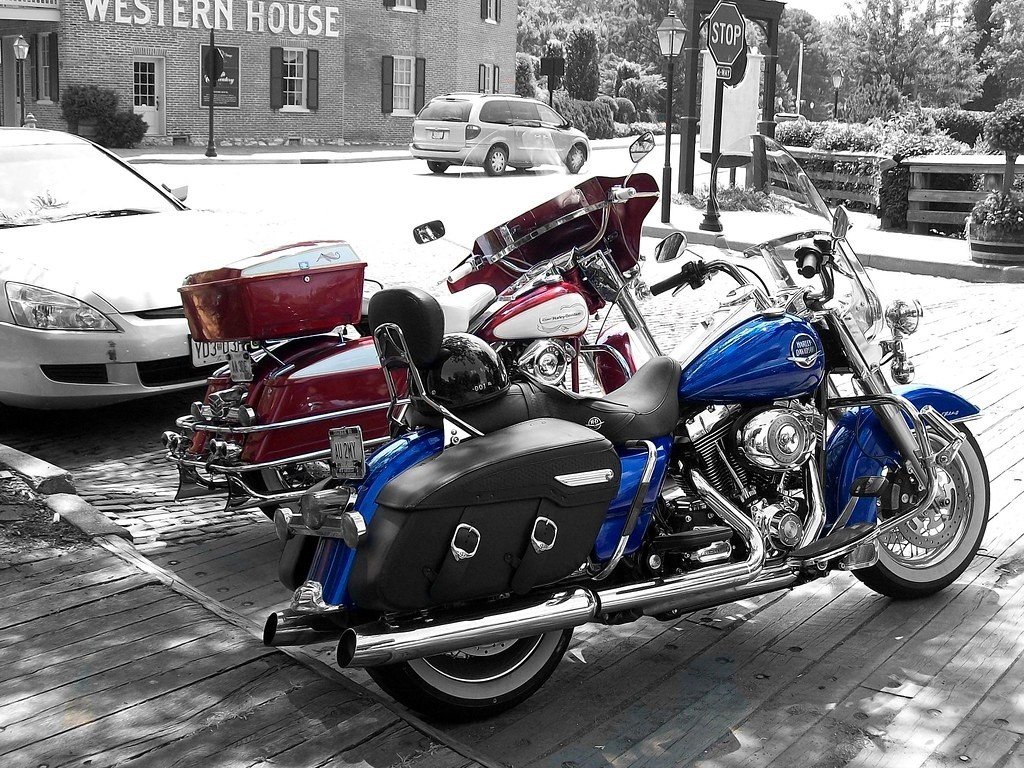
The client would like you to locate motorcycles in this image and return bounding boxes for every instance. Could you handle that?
[262,133,990,721]
[163,117,666,522]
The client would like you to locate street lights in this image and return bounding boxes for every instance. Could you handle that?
[12,36,30,126]
[657,11,689,223]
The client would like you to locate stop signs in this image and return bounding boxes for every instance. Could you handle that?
[707,1,745,65]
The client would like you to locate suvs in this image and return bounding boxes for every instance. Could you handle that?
[408,90,590,177]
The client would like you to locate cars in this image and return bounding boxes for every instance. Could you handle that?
[1,129,278,411]
